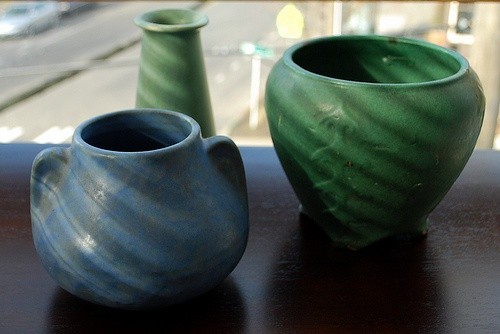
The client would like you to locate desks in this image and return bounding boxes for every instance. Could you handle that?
[0,142,500,334]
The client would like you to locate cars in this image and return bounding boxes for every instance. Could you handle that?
[0,1,96,38]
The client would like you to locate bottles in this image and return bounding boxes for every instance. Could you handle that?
[134,6,217,138]
